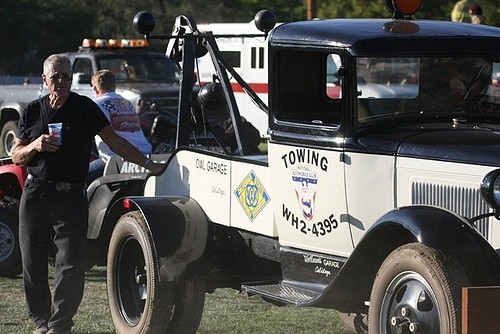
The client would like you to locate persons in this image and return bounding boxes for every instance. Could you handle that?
[11,54,168,334]
[87,69,153,185]
[451,0,487,24]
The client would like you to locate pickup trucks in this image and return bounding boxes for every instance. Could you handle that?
[0,38,204,162]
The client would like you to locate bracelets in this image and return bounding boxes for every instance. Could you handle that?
[144,159,153,169]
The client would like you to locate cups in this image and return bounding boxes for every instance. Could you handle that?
[48,123,63,145]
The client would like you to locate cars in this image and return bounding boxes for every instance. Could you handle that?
[90,11,500,333]
[190,21,500,135]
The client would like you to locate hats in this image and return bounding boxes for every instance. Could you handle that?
[468,5,483,15]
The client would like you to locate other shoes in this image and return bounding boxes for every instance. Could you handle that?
[31,322,71,333]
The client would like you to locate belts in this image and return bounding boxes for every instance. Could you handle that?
[26,174,86,193]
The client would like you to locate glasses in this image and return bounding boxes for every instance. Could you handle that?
[44,73,73,81]
